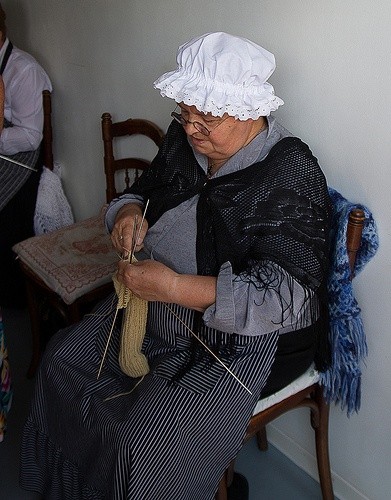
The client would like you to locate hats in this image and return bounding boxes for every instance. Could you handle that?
[152,32,284,121]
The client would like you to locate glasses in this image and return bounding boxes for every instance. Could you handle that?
[171,103,233,136]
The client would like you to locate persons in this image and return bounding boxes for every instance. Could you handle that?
[0,6,53,307]
[20,32,330,499]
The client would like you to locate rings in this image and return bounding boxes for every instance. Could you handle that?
[118,236,123,240]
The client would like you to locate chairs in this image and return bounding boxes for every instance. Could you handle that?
[216,187,366,500]
[13,89,168,378]
